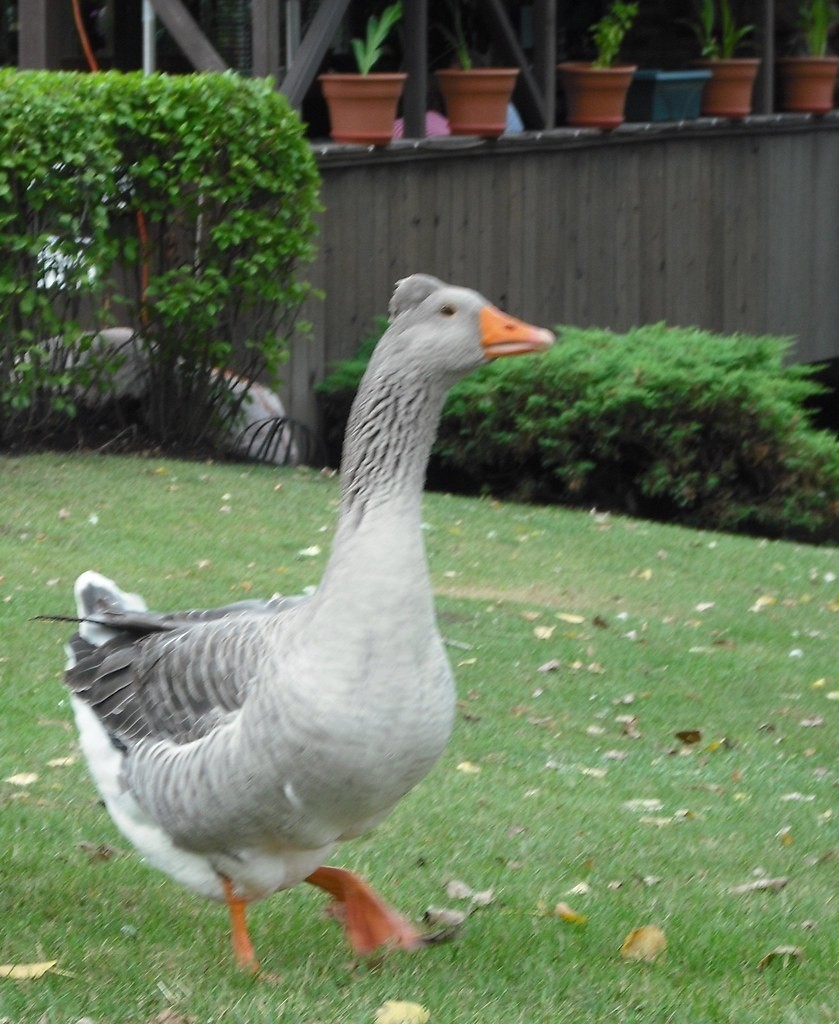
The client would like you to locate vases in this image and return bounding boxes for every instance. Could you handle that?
[625,70,712,123]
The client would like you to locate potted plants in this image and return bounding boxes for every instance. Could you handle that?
[681,0,761,117]
[557,2,636,127]
[319,4,408,145]
[775,0,839,113]
[435,6,519,138]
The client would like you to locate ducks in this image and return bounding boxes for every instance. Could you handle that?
[24,273,556,984]
